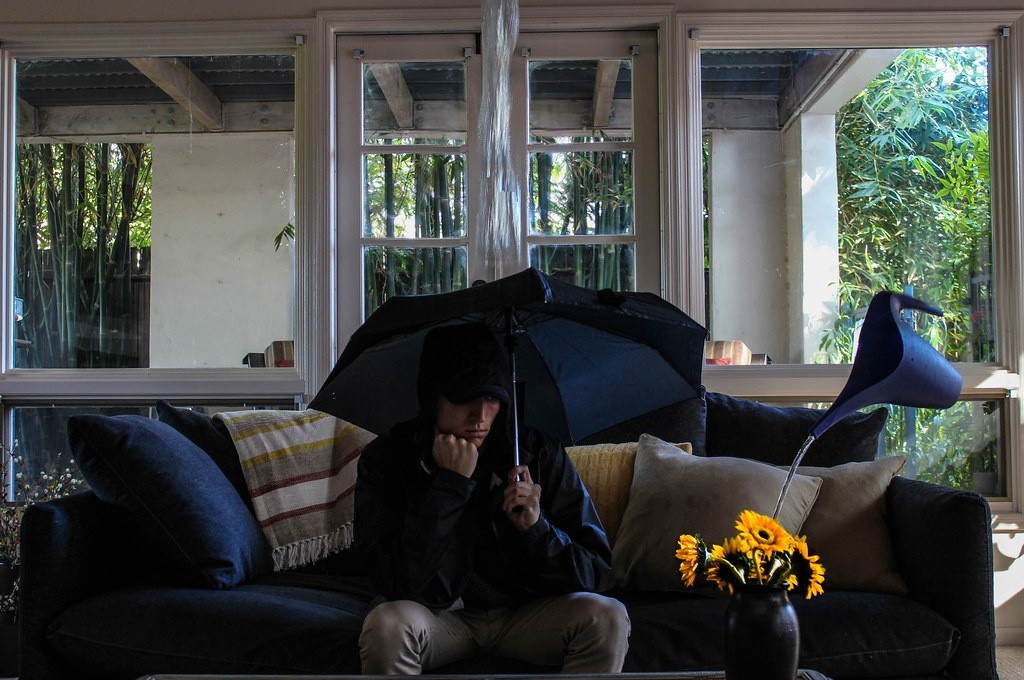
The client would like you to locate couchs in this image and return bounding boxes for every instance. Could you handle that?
[16,476,1003,680]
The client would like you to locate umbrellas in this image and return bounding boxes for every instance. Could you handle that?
[302,262,713,515]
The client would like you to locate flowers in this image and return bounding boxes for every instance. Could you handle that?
[673,505,827,600]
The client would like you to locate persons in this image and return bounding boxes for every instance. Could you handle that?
[350,322,636,678]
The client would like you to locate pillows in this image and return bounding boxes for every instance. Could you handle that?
[64,410,274,585]
[565,434,694,537]
[773,454,910,597]
[581,382,707,458]
[604,433,824,592]
[157,398,250,503]
[703,386,888,474]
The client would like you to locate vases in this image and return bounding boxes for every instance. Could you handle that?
[721,585,802,680]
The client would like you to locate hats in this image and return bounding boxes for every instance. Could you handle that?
[417,326,514,403]
